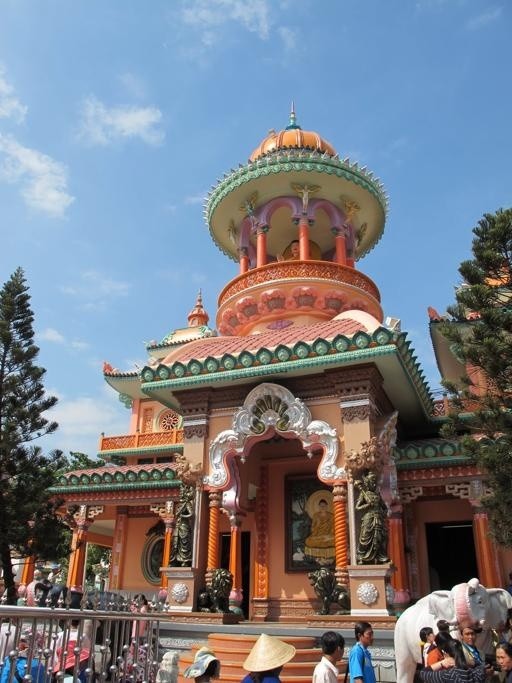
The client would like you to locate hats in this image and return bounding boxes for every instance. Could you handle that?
[458,622,482,633]
[52,641,89,672]
[244,632,297,673]
[184,647,220,679]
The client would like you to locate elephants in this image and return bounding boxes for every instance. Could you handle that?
[391,575,512,682]
[32,578,131,681]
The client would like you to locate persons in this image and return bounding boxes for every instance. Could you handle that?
[277,240,300,262]
[241,633,296,682]
[311,621,378,682]
[168,490,194,567]
[305,500,335,549]
[0,565,164,682]
[355,471,390,564]
[185,648,222,683]
[419,620,511,682]
